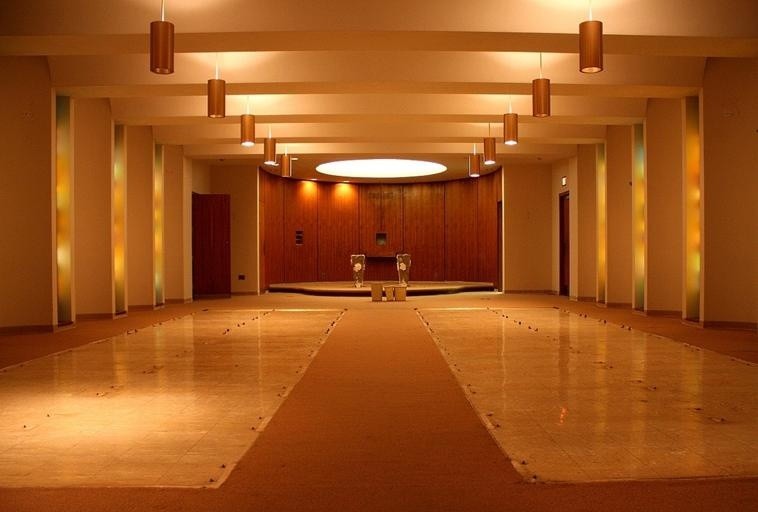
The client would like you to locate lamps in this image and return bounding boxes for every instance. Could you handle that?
[469,1,603,178]
[150,0,291,178]
